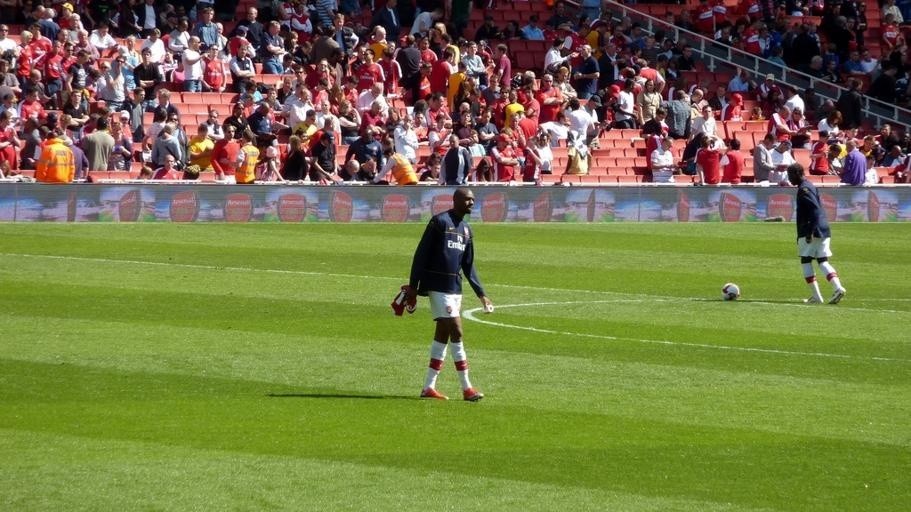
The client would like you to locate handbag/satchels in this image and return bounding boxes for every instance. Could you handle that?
[174,160,185,170]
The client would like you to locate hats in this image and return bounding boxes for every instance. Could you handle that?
[62,2,73,11]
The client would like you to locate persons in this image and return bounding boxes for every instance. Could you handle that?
[1,1,910,184]
[407,188,491,399]
[788,164,846,305]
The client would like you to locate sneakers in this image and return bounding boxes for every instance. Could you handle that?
[463,386,484,400]
[420,386,449,400]
[802,296,824,304]
[827,287,846,304]
